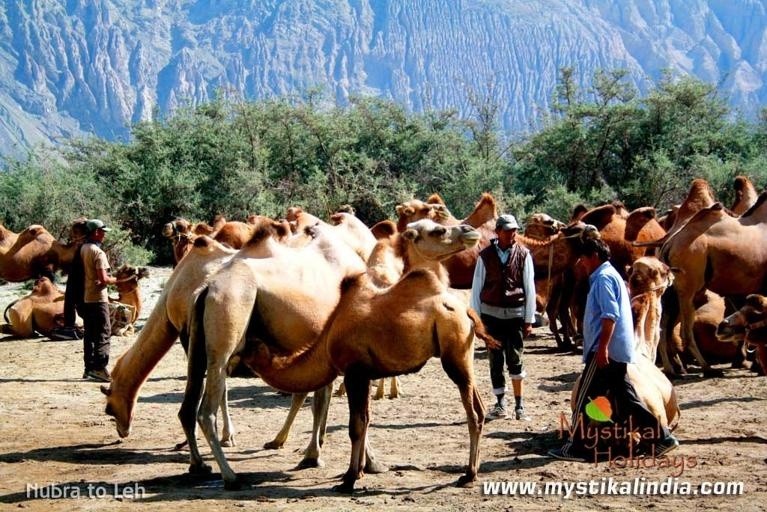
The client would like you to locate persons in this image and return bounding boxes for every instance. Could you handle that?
[547,222,678,462]
[78,219,129,381]
[470,214,537,420]
[54,221,91,341]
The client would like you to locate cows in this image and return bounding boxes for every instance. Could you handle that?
[99,176,766,498]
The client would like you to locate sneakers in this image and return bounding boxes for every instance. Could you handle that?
[643,434,680,459]
[83,366,113,383]
[548,443,586,462]
[485,403,509,420]
[514,405,526,420]
[52,328,76,340]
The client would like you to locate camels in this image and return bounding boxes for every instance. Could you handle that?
[99,176,766,498]
[315,215,322,217]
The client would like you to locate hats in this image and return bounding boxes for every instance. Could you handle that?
[495,214,520,231]
[84,219,112,232]
